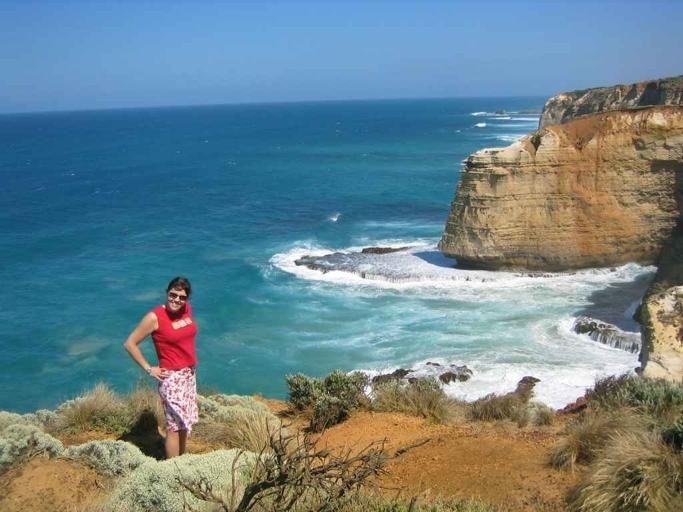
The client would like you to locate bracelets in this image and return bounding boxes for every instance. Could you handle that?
[146,366,152,374]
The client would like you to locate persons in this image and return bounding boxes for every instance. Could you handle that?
[124,277,197,459]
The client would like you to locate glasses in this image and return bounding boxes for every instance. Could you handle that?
[168,291,187,301]
